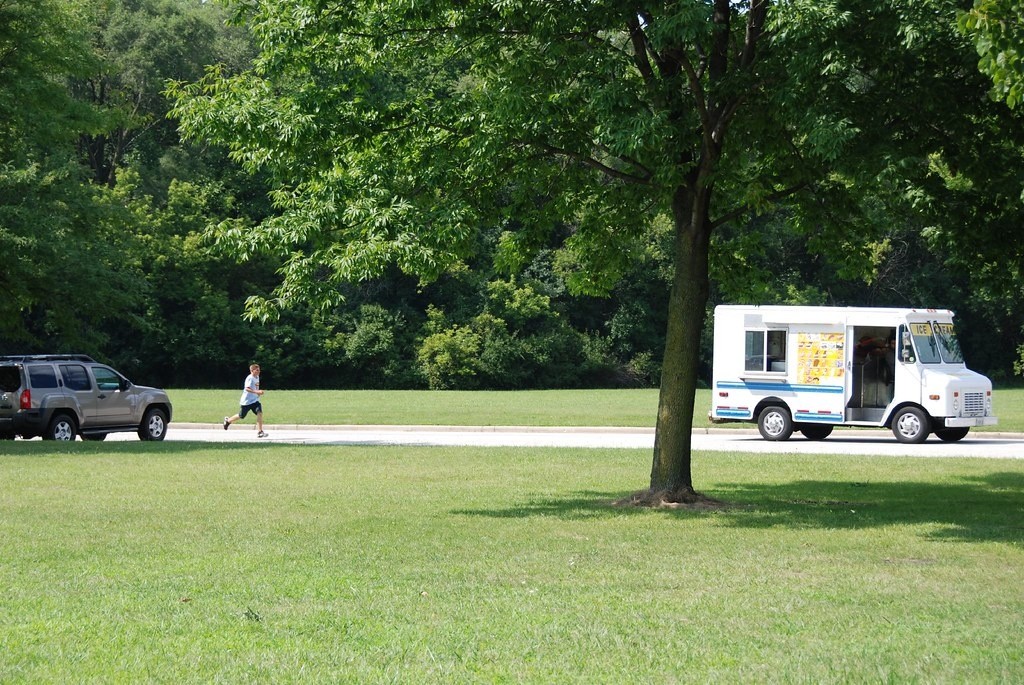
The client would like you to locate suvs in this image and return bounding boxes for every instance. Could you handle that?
[0,354,174,446]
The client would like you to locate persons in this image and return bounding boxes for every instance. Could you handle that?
[224,364,268,438]
[886,337,896,376]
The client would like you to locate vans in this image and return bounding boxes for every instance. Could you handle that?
[708,304,999,444]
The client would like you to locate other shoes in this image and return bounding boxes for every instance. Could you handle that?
[223,416,230,431]
[257,429,269,438]
